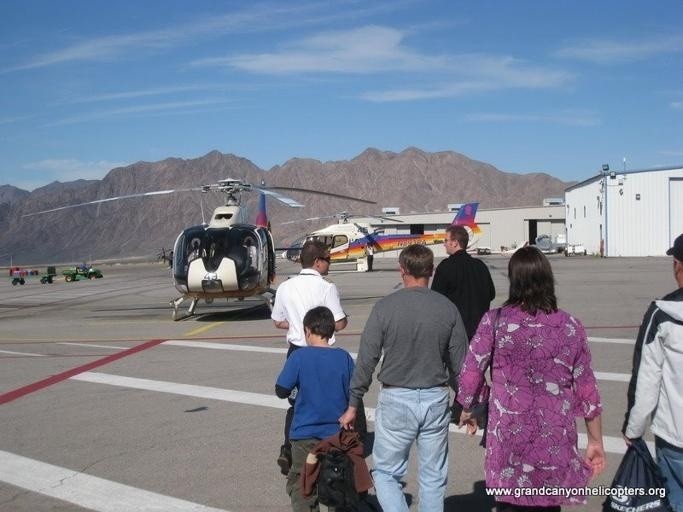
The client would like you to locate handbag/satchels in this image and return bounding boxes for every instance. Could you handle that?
[318,452,357,507]
[471,403,489,447]
[602,438,672,512]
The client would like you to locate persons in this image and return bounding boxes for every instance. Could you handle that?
[431,226,495,424]
[167,251,174,269]
[338,244,470,512]
[621,234,683,511]
[272,241,347,471]
[276,306,366,512]
[365,243,375,271]
[454,246,605,512]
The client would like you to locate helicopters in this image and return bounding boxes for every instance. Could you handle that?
[281,202,486,273]
[22,177,376,322]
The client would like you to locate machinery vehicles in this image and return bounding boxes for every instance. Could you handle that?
[63,265,103,281]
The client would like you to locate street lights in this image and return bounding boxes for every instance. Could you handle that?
[602,164,615,257]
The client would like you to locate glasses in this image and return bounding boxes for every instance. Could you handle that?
[313,256,330,263]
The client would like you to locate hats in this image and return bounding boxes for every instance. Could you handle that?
[666,234,683,262]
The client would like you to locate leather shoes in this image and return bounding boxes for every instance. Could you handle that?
[278,448,291,476]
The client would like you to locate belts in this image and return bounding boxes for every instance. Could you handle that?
[382,383,449,388]
